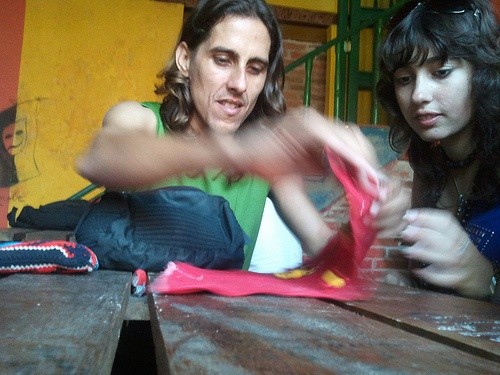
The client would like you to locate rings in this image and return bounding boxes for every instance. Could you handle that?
[345,124,350,129]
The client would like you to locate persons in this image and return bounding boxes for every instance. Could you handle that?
[375,0,500,306]
[73,0,410,273]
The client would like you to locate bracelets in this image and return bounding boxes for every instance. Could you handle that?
[483,261,500,305]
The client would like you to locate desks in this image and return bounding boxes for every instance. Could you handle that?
[1,267,500,375]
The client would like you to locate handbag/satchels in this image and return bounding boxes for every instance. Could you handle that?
[75,180,245,269]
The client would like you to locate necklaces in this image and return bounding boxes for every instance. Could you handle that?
[452,176,472,216]
[437,146,479,170]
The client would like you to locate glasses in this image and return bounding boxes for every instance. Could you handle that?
[386,1,479,29]
